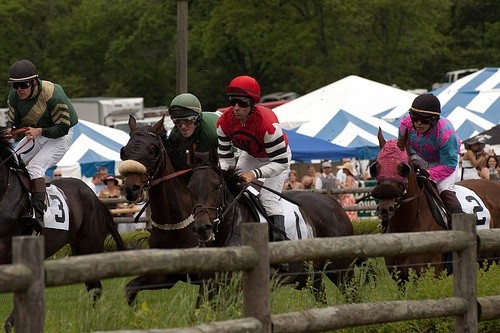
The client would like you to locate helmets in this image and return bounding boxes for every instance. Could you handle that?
[8,60,39,83]
[226,76,261,104]
[409,93,441,122]
[168,93,201,123]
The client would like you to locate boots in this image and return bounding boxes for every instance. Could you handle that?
[29,177,46,232]
[439,190,463,214]
[268,215,289,272]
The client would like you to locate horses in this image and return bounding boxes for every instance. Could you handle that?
[0,126,149,333]
[186,140,378,305]
[370,127,500,293]
[120,114,220,309]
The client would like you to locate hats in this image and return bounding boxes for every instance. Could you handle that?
[322,162,331,167]
[464,138,485,153]
[336,162,354,176]
[103,176,118,186]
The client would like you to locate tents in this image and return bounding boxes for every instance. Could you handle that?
[12,119,148,232]
[271,68,500,164]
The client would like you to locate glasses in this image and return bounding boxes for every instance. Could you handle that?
[171,115,199,126]
[13,80,34,89]
[228,98,252,108]
[411,115,433,124]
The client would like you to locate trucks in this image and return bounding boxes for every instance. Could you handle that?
[69,97,157,135]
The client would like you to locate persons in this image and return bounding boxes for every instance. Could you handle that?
[2,60,78,232]
[87,172,107,195]
[316,158,371,181]
[315,162,341,201]
[398,94,464,214]
[459,152,500,182]
[461,140,494,181]
[53,170,62,180]
[336,162,357,221]
[97,176,126,229]
[284,171,304,190]
[167,93,244,168]
[99,167,109,178]
[216,76,292,273]
[301,165,322,189]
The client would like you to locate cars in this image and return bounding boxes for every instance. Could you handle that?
[432,69,478,91]
